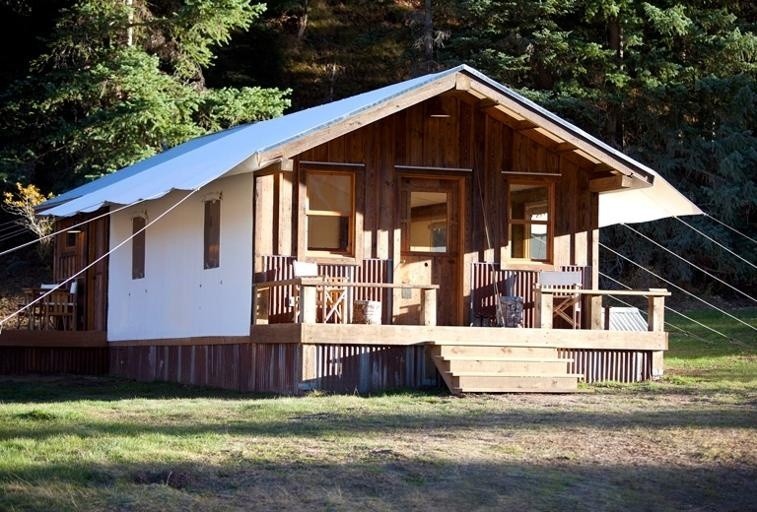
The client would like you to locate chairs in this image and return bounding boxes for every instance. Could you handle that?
[290,258,350,324]
[14,277,80,333]
[534,270,583,327]
